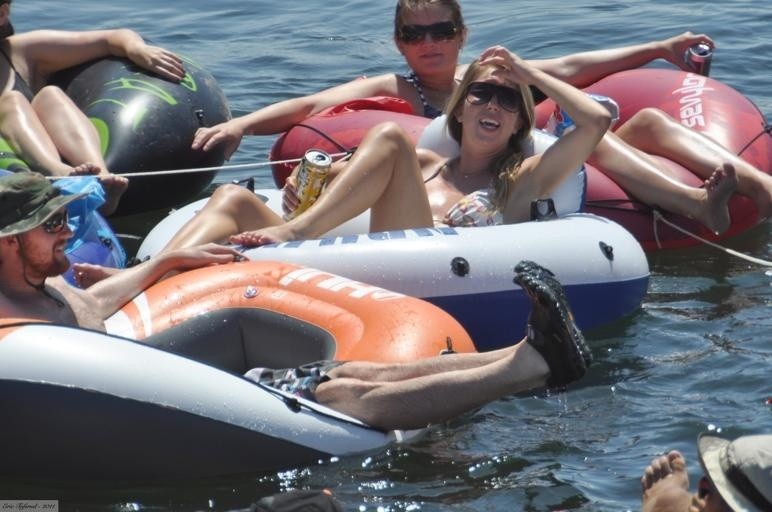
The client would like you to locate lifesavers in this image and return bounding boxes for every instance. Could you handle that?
[1,259,483,490]
[131,179,650,355]
[270,68,770,252]
[0,44,230,232]
[0,168,127,290]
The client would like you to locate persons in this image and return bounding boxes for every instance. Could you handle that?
[1,1,186,218]
[638,429,772,511]
[192,0,772,236]
[68,48,609,290]
[0,172,592,430]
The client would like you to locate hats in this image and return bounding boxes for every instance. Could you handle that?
[696,431,772,512]
[0,170,90,240]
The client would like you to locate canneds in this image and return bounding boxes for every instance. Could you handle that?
[282,150,332,221]
[687,43,712,77]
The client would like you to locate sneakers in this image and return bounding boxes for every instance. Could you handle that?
[511,257,594,389]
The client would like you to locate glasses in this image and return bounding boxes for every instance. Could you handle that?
[396,19,464,43]
[697,474,724,503]
[461,81,525,115]
[38,208,69,234]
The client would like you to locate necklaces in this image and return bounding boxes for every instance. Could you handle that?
[454,162,490,179]
